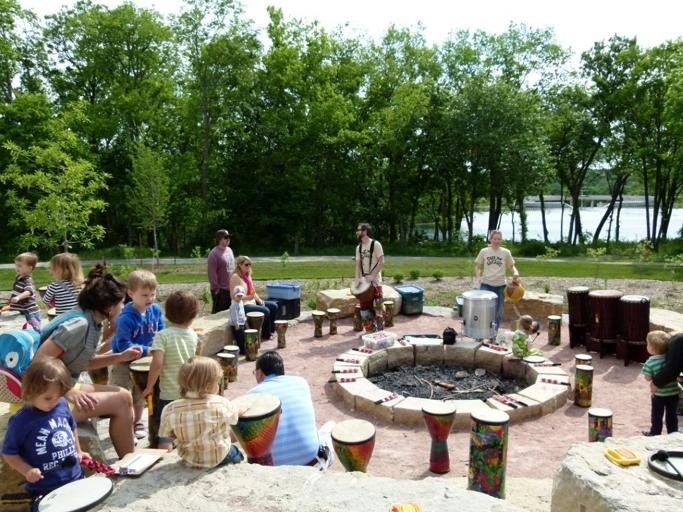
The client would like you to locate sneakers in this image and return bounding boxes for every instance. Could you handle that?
[134,417,146,437]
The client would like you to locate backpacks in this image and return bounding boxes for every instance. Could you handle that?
[0,311,91,403]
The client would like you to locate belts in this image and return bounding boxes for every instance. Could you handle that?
[305,456,321,466]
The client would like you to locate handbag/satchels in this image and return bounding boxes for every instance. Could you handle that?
[443,326,458,345]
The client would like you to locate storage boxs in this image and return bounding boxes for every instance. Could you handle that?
[263,282,302,320]
[395,284,424,317]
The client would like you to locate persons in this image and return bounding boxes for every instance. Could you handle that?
[227,254,279,339]
[226,284,249,356]
[649,332,683,397]
[205,229,237,313]
[1,248,242,512]
[639,328,678,438]
[472,231,524,346]
[246,348,335,473]
[510,313,542,359]
[351,221,385,333]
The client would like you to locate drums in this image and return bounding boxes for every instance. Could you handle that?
[229,395,281,465]
[275,321,289,349]
[245,312,265,361]
[129,358,160,448]
[588,408,613,442]
[422,406,456,474]
[568,286,589,349]
[350,277,394,336]
[216,345,240,396]
[38,286,55,307]
[312,310,325,337]
[467,410,509,498]
[547,315,562,346]
[326,307,339,335]
[589,289,622,358]
[331,419,376,473]
[619,296,651,365]
[47,307,56,321]
[574,353,594,407]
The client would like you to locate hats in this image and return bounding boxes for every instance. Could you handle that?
[215,230,233,238]
[233,286,245,298]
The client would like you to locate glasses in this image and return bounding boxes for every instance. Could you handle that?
[243,264,251,267]
